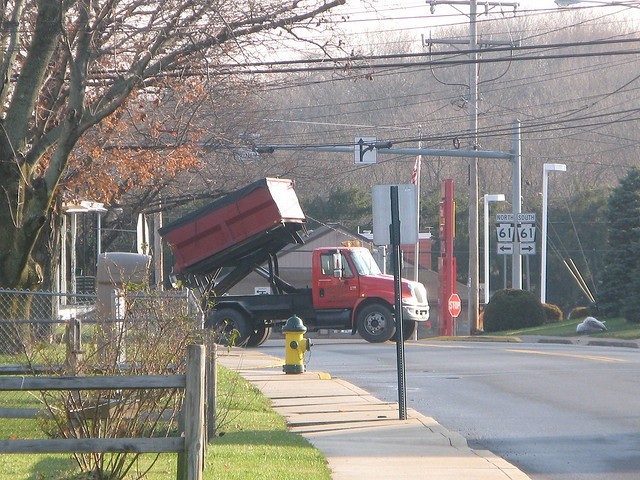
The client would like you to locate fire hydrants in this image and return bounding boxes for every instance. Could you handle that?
[282,314,315,374]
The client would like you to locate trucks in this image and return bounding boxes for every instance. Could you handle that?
[158,177,430,347]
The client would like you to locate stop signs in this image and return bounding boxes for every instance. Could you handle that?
[448,294,461,319]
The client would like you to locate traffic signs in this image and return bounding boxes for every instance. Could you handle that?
[497,212,514,222]
[516,213,535,224]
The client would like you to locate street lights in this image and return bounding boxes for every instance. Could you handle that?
[541,164,566,304]
[553,0,639,17]
[483,194,506,304]
